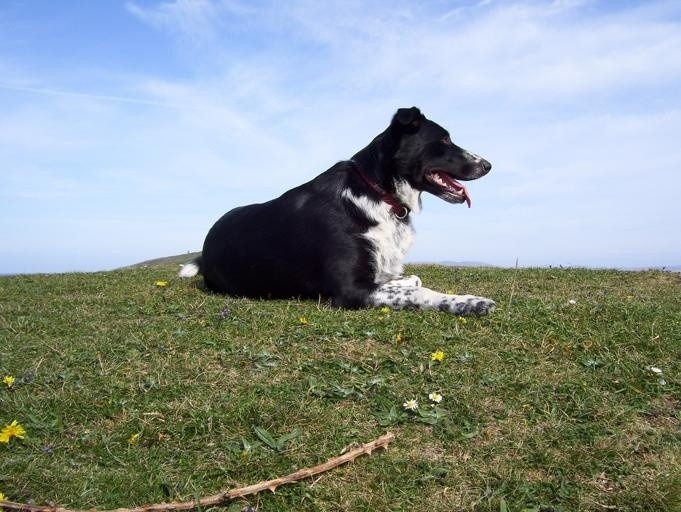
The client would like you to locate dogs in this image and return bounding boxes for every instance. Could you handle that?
[177,106,496,317]
